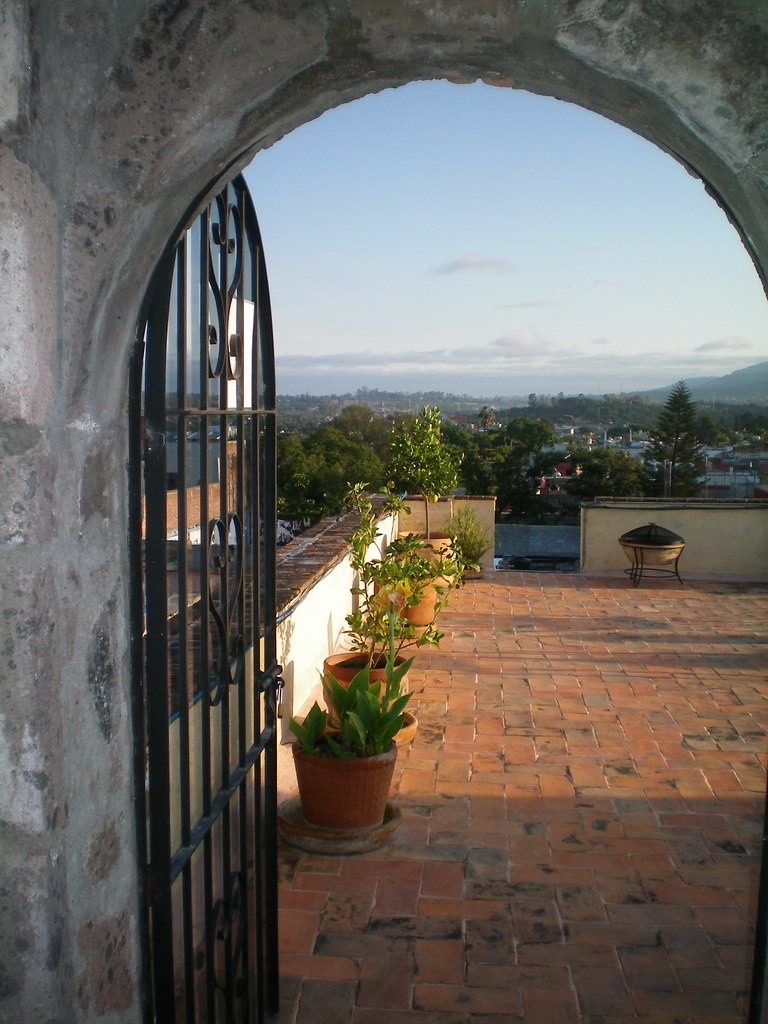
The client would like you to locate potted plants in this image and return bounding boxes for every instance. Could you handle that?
[284,660,419,829]
[363,531,436,626]
[322,605,408,729]
[381,404,459,588]
[446,495,496,579]
